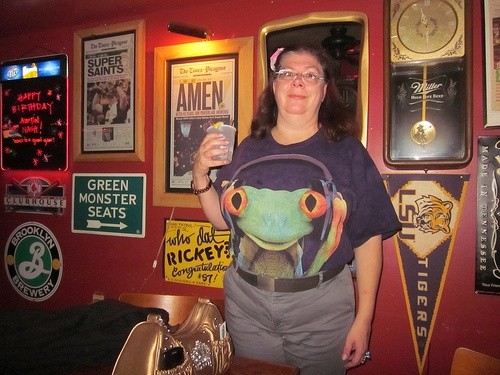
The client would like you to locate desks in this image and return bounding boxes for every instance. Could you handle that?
[61,355,292,375]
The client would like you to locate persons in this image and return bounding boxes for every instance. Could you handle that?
[90,81,130,125]
[192,42,402,375]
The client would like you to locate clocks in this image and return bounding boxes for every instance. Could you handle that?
[381,0,473,171]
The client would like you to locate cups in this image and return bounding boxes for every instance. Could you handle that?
[207,125,236,164]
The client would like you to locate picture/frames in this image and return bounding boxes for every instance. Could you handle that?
[71,172,146,238]
[5,221,64,302]
[153,35,254,209]
[72,19,147,163]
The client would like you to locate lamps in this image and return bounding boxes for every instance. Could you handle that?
[166,22,207,39]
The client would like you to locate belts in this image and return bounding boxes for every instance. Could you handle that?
[232,259,346,293]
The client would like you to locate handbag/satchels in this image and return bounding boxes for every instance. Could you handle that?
[110,295,235,375]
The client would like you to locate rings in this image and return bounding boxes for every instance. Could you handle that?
[360,352,370,363]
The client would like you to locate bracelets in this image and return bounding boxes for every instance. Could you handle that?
[191,176,212,195]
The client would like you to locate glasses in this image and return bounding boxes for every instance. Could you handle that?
[274,69,329,82]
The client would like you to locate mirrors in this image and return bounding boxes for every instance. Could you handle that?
[256,10,370,148]
